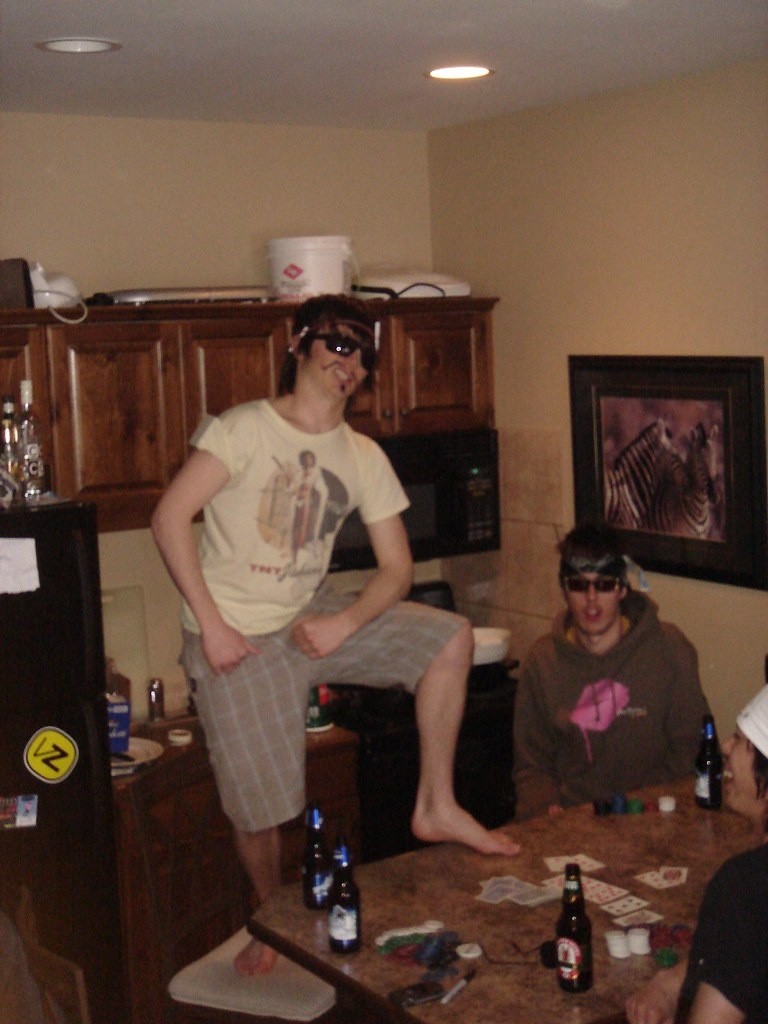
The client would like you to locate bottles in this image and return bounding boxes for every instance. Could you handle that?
[105,658,130,754]
[327,834,360,954]
[556,863,593,993]
[694,715,723,809]
[1,379,50,494]
[303,799,333,910]
[147,678,166,725]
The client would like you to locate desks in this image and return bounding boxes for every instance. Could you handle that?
[249,767,767,1023]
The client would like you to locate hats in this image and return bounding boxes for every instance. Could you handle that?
[735,684,768,758]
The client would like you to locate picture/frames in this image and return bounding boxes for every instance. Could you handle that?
[567,352,766,595]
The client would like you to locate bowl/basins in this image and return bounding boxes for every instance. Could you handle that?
[472,625,512,666]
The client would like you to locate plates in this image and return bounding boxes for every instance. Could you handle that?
[111,736,162,767]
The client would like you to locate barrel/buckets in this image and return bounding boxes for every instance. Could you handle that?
[259,236,353,301]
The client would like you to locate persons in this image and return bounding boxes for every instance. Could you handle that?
[624,687,768,1024]
[152,291,521,973]
[509,522,717,822]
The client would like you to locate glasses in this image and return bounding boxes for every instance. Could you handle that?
[564,578,620,592]
[306,332,380,374]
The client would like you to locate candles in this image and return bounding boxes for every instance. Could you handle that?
[20,374,36,411]
[4,391,15,417]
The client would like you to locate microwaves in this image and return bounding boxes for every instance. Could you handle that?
[327,429,501,572]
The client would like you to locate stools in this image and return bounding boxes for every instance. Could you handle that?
[166,927,336,1024]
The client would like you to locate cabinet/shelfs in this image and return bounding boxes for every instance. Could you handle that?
[299,297,505,433]
[54,309,287,534]
[110,729,369,1023]
[1,308,49,507]
